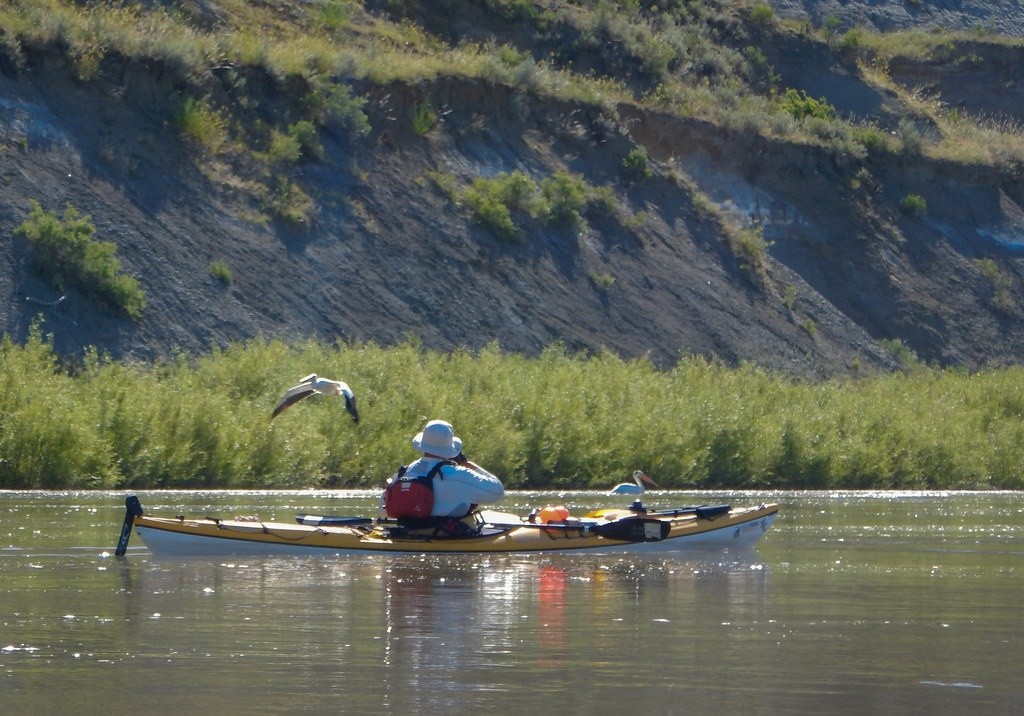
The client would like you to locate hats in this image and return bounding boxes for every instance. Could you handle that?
[412,420,462,458]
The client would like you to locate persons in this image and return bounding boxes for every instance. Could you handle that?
[384,420,504,540]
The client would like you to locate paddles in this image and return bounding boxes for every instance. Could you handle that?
[294,514,671,542]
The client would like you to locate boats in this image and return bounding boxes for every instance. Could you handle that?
[119,494,779,560]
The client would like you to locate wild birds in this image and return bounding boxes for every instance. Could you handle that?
[608,470,659,493]
[269,372,361,427]
